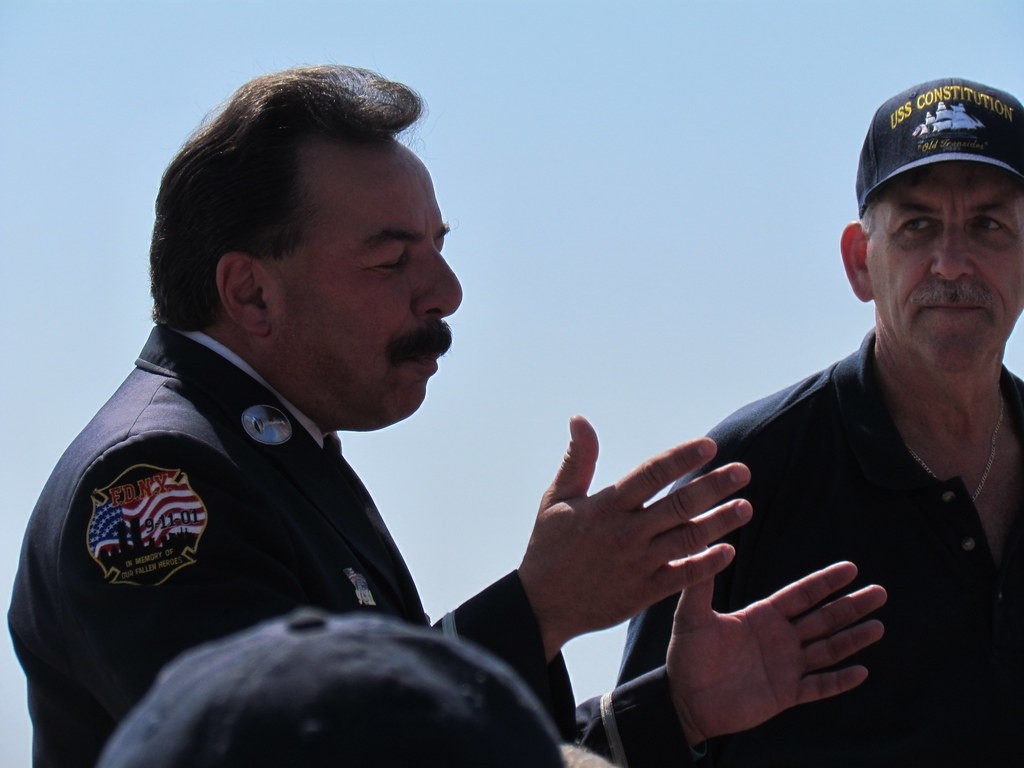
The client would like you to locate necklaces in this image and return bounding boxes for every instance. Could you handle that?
[903,397,1006,504]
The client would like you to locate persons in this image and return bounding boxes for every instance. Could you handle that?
[96,606,567,768]
[6,65,886,768]
[617,76,1024,768]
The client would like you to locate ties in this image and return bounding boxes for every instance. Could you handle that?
[322,435,343,457]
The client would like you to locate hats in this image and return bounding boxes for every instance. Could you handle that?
[856,77,1024,217]
[99,607,569,768]
